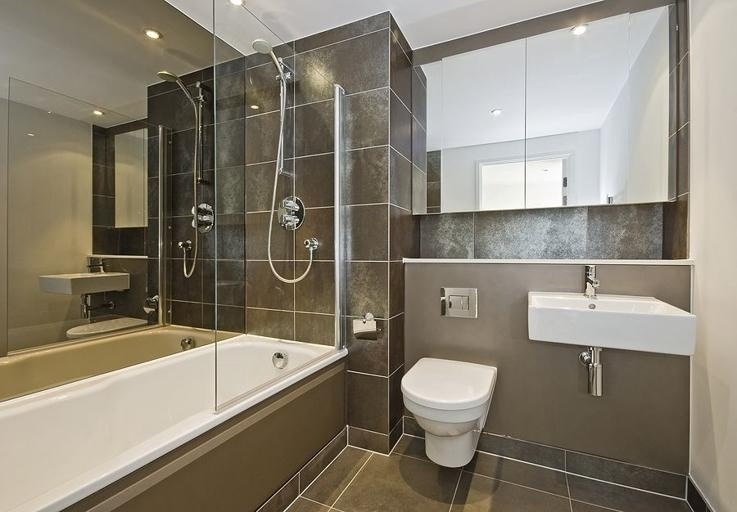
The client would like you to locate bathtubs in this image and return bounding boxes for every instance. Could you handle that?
[1,335,348,512]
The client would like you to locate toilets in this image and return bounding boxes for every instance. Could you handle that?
[400,358,498,470]
[65,319,150,337]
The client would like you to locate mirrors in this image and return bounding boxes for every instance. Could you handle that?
[409,1,681,218]
[0,1,243,402]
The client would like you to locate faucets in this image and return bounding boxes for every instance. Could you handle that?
[86,262,102,270]
[583,265,600,298]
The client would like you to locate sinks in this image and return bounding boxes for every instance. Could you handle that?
[38,273,131,296]
[528,293,697,358]
